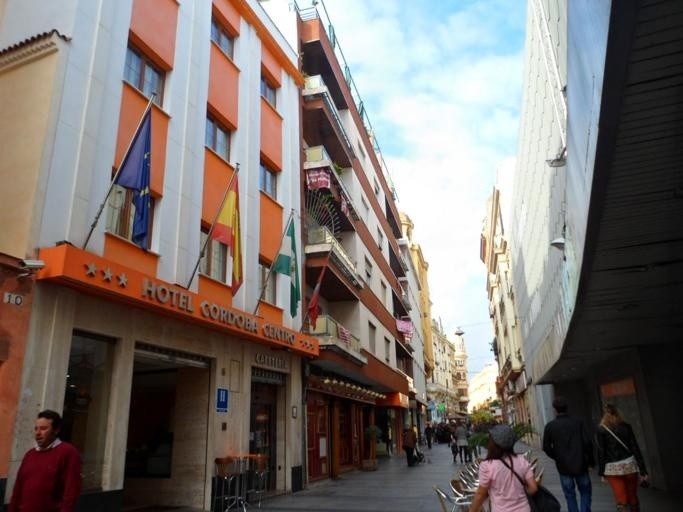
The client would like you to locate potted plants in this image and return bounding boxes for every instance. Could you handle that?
[363,426,382,471]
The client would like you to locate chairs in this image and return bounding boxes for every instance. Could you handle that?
[432,449,544,512]
[215,453,270,512]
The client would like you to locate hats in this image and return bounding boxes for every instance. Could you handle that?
[488,424,513,448]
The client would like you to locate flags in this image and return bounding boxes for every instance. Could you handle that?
[210,174,243,298]
[114,108,152,251]
[270,216,300,318]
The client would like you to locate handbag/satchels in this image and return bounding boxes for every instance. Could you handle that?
[529,485,561,512]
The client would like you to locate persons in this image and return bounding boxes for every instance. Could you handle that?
[7,409,83,512]
[597,401,649,512]
[424,421,482,463]
[543,396,598,512]
[468,425,538,512]
[401,427,417,468]
